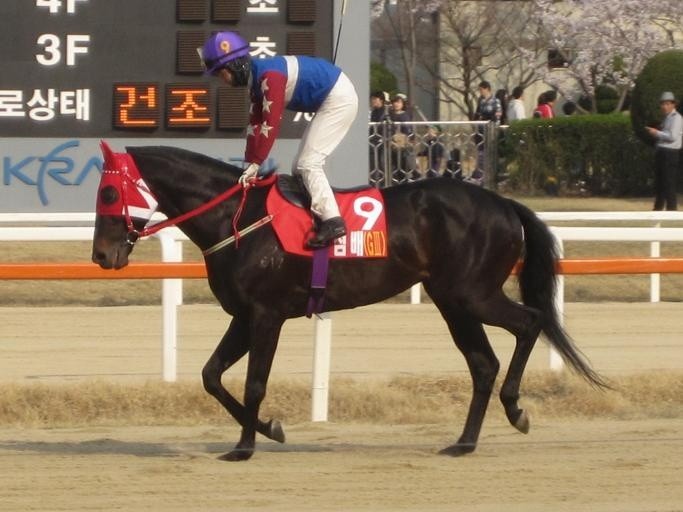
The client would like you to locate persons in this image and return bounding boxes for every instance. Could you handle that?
[369,89,384,190]
[416,80,581,187]
[386,93,419,187]
[648,91,682,214]
[197,27,358,248]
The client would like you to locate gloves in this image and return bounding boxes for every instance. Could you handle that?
[238,163,259,188]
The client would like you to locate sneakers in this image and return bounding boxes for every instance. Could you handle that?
[310,217,345,247]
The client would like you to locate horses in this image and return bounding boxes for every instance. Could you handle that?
[92,138,619,462]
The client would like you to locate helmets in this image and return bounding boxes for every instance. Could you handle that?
[202,31,249,73]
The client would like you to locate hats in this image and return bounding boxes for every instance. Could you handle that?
[659,91,680,107]
